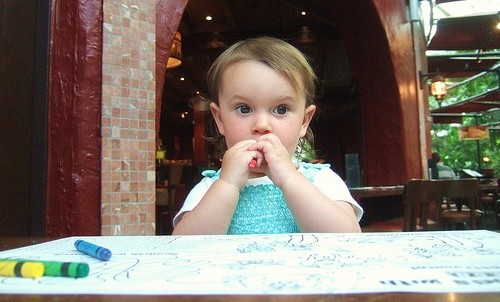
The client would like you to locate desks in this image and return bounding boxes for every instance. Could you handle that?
[0,228,500,302]
[455,179,499,225]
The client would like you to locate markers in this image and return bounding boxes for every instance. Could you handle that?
[247,157,258,170]
[73,238,112,261]
[0,258,90,279]
[73,238,111,260]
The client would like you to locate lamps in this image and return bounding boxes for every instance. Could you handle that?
[460,126,490,141]
[418,69,446,107]
[166,31,182,69]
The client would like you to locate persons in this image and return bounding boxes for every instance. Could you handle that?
[172,37,363,235]
[428,153,456,179]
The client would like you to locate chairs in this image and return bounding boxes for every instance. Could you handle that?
[478,169,496,220]
[402,178,479,232]
